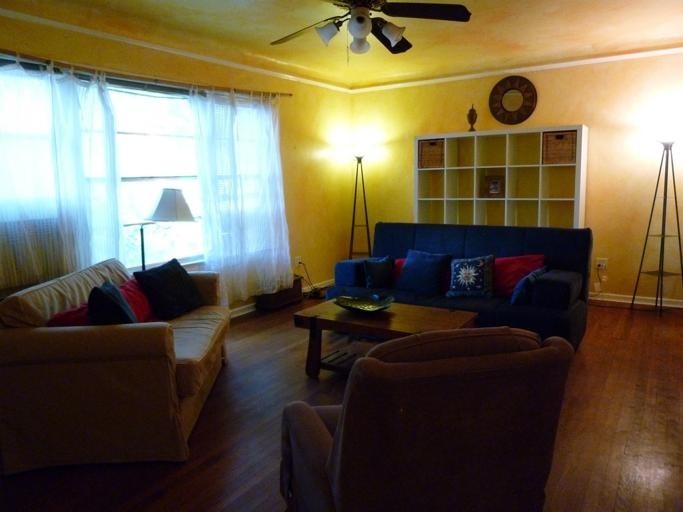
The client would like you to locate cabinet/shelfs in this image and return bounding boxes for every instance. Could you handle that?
[413,125,586,229]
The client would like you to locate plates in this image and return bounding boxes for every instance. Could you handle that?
[332,295,393,315]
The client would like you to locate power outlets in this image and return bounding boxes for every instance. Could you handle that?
[596,257,608,268]
[295,256,303,267]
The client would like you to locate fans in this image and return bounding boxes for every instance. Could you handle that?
[271,0,472,57]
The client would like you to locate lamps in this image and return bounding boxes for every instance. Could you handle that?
[315,8,406,54]
[631,122,683,312]
[139,190,194,269]
[343,134,372,261]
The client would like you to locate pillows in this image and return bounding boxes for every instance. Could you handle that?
[493,252,541,296]
[512,263,546,303]
[447,256,494,300]
[363,254,397,289]
[134,262,204,318]
[88,280,138,326]
[396,250,448,296]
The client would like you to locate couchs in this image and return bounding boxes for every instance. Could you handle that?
[281,329,574,512]
[0,259,228,479]
[325,223,592,355]
